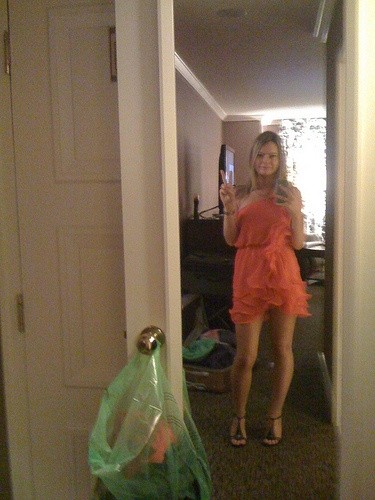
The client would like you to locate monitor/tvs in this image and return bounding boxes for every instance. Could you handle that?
[219,144,236,214]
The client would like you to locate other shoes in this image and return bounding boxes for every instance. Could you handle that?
[261,415,283,447]
[228,413,248,448]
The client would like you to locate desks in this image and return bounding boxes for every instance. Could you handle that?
[182,249,241,328]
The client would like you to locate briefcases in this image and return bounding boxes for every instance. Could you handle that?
[181,294,237,393]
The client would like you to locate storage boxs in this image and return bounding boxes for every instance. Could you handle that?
[181,360,238,392]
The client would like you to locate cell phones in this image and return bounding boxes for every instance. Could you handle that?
[274,179,289,205]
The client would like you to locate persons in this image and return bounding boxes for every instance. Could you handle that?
[220,131,312,446]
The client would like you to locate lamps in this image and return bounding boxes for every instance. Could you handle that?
[313,0,331,44]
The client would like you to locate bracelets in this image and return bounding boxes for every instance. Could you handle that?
[223,207,238,217]
